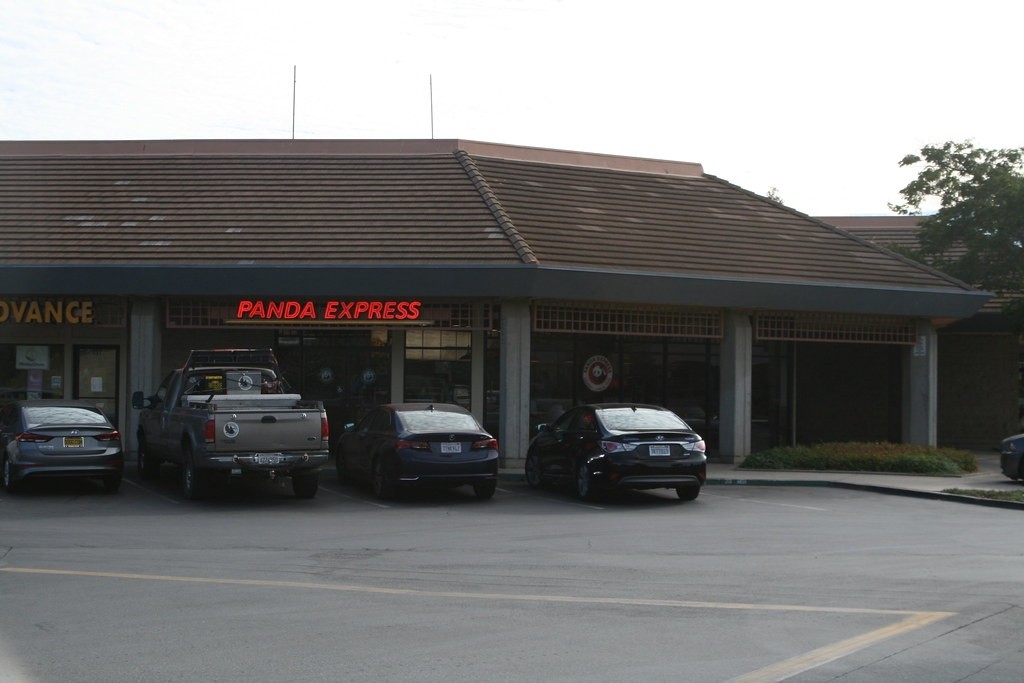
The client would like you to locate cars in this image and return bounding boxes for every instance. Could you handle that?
[1000,434,1024,482]
[0,399,124,497]
[406,384,500,411]
[335,401,499,502]
[526,403,706,503]
[528,397,581,439]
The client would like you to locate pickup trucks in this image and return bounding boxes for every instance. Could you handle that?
[133,363,331,502]
